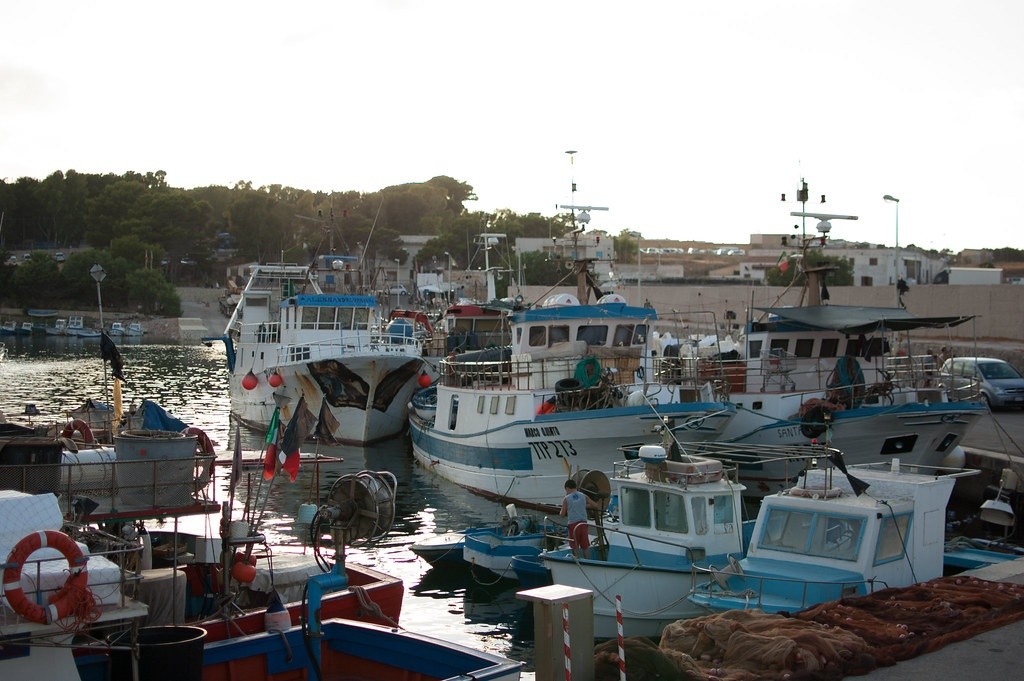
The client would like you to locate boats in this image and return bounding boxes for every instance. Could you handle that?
[0,320,18,334]
[689,454,1023,626]
[124,321,144,336]
[13,320,34,334]
[30,319,49,332]
[521,404,757,642]
[507,555,559,594]
[0,262,528,681]
[403,205,744,519]
[460,522,568,582]
[75,328,102,337]
[108,322,126,336]
[620,174,990,491]
[62,315,86,335]
[219,252,434,446]
[409,525,481,569]
[45,318,67,335]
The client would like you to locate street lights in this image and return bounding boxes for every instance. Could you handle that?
[882,194,902,358]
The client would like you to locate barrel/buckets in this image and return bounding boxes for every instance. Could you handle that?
[113,433,198,508]
[105,625,208,680]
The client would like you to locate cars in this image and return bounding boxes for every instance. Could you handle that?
[937,356,1024,411]
[638,246,744,257]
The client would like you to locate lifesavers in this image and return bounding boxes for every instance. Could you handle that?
[3,531,88,624]
[62,419,93,444]
[179,427,216,492]
[801,412,825,438]
[808,407,832,432]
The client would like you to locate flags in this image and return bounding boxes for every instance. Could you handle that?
[100,332,127,383]
[821,279,829,301]
[777,251,791,273]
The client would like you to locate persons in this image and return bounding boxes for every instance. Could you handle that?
[560,480,599,559]
[923,348,937,388]
[938,346,950,367]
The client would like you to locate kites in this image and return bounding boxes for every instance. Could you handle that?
[263,390,319,481]
